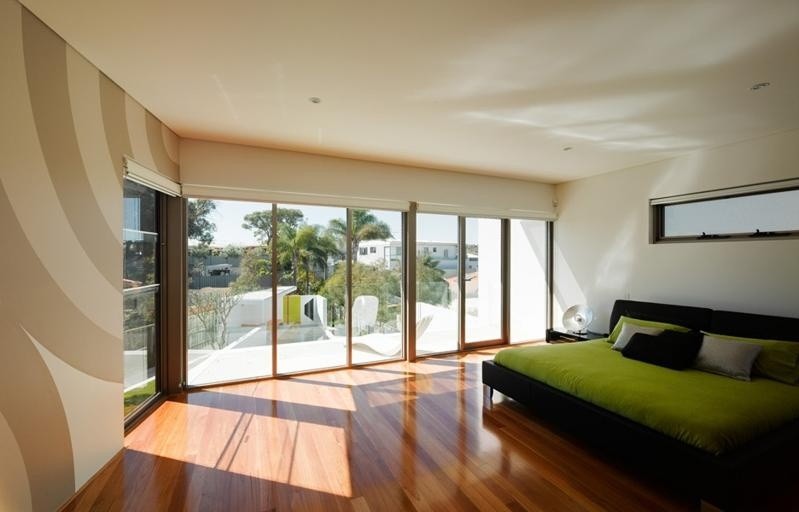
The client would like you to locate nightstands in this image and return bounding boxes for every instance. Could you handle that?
[545,325,608,344]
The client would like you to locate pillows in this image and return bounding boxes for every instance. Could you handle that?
[608,313,799,387]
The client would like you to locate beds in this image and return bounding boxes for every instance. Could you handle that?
[482,300,799,498]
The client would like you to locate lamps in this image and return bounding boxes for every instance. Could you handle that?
[563,303,594,335]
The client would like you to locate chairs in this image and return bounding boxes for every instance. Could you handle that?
[345,293,435,353]
